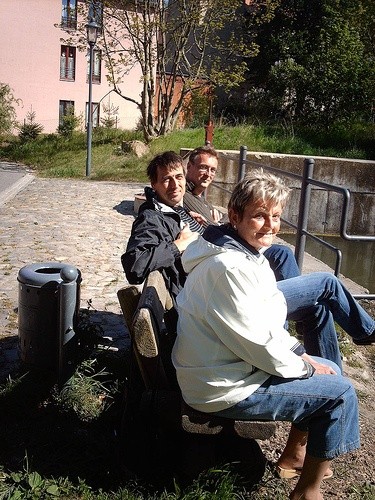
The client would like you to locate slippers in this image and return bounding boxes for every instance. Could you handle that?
[276,465,333,479]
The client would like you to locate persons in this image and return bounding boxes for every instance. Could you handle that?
[182,146,300,280]
[172,167,361,500]
[121,151,375,375]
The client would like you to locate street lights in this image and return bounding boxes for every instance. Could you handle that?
[84,15,102,178]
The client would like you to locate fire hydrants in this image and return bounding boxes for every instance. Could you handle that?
[205,119,215,145]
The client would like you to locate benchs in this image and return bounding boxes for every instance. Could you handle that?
[118,270,276,440]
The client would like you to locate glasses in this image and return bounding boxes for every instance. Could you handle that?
[192,162,218,174]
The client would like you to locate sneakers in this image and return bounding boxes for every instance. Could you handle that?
[295,322,303,335]
[352,329,375,345]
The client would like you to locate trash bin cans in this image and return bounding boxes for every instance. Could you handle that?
[17,261,82,369]
